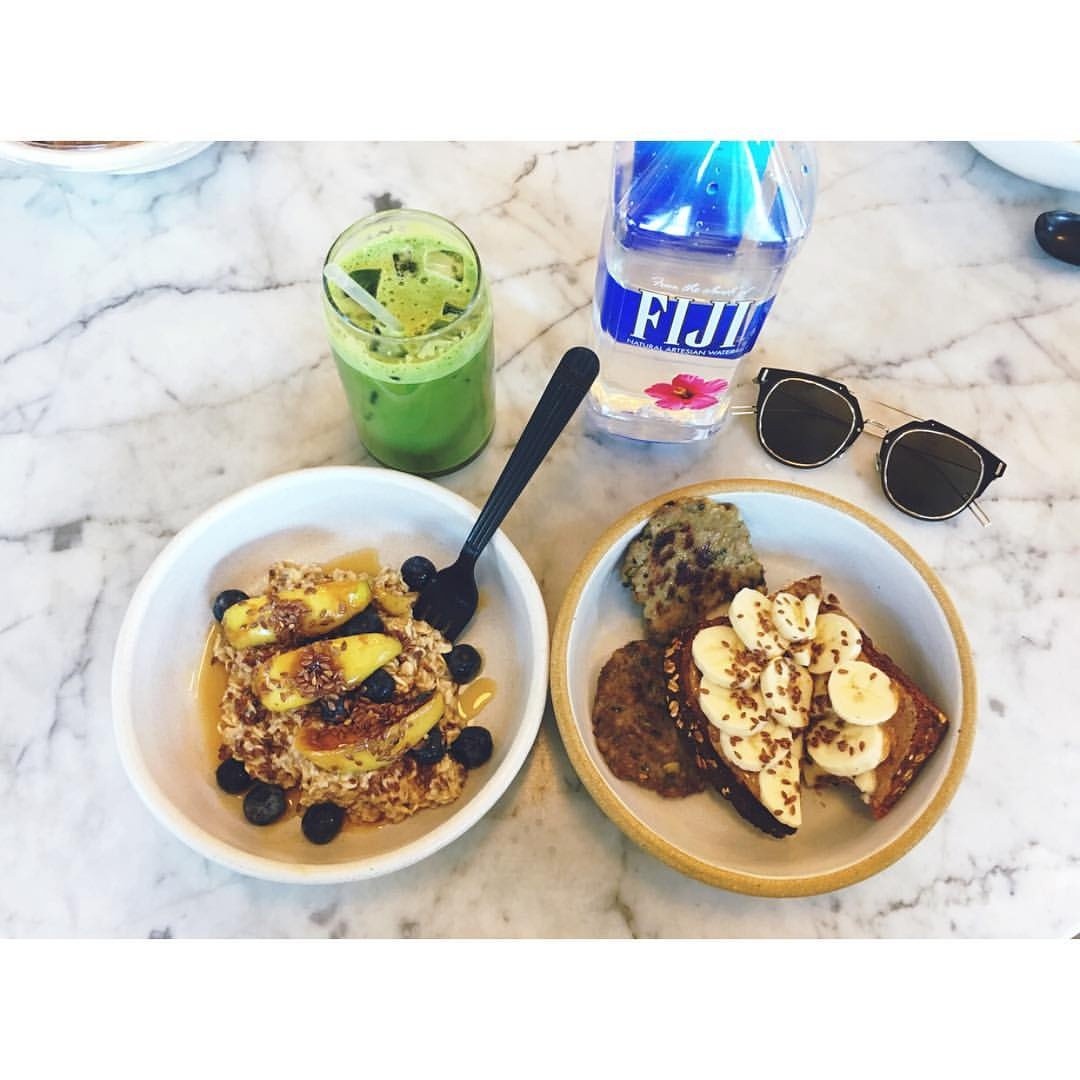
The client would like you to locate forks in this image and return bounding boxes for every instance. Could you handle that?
[408,345,600,642]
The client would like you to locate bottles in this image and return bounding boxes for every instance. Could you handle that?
[578,141,823,472]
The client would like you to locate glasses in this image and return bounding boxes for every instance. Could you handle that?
[751,365,1009,528]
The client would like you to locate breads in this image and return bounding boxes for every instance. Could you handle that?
[661,566,826,833]
[794,589,945,826]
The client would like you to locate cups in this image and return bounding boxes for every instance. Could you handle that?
[316,205,497,479]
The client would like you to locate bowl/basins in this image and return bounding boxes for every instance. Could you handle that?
[112,464,550,884]
[552,477,977,901]
